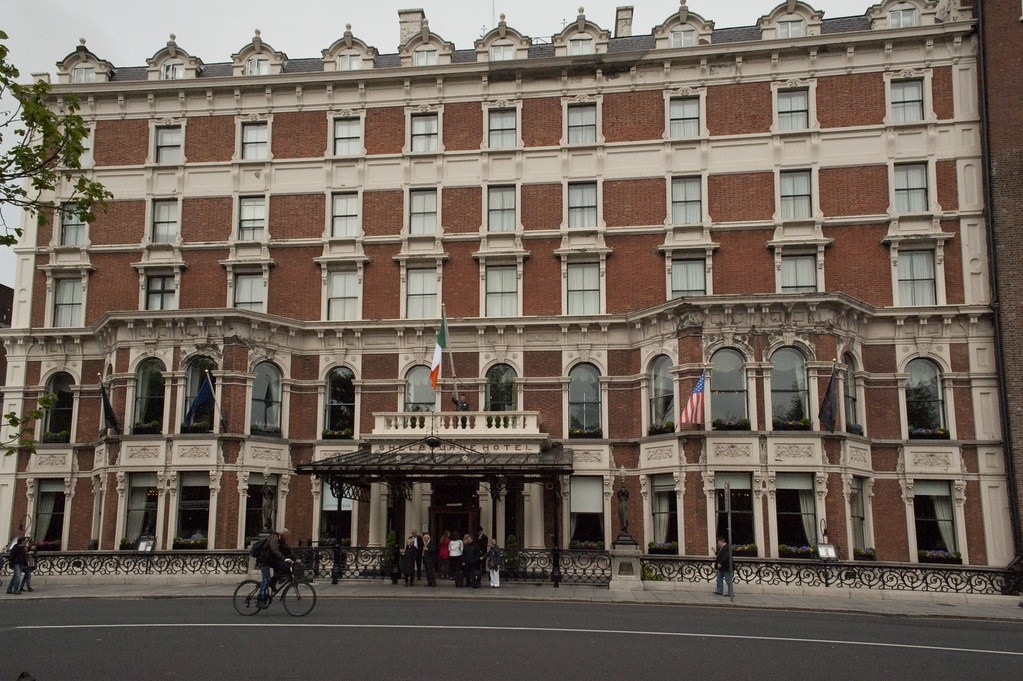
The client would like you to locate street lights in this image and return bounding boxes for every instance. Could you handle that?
[613,463,639,549]
[261,464,275,536]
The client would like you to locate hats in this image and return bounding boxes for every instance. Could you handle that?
[475,526,483,532]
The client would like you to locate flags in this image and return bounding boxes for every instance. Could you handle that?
[679,370,705,424]
[429,316,446,390]
[818,365,837,434]
[184,379,211,423]
[101,385,119,429]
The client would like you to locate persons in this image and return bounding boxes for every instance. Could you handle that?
[401,539,417,586]
[408,529,424,581]
[438,525,488,588]
[261,484,275,529]
[451,391,469,429]
[711,537,734,597]
[255,528,301,609]
[479,539,501,589]
[422,534,437,587]
[191,529,203,540]
[5,537,34,595]
[616,484,631,533]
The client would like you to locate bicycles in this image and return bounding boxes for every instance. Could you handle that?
[233,561,316,617]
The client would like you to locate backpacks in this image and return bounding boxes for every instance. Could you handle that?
[250,532,279,558]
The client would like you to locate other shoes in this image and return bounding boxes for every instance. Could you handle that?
[490,586,497,589]
[723,594,734,597]
[19,587,26,591]
[713,591,722,595]
[425,582,435,586]
[14,589,21,594]
[256,595,268,609]
[410,583,414,586]
[464,584,472,587]
[267,578,278,593]
[6,590,13,594]
[405,582,408,586]
[28,588,34,592]
